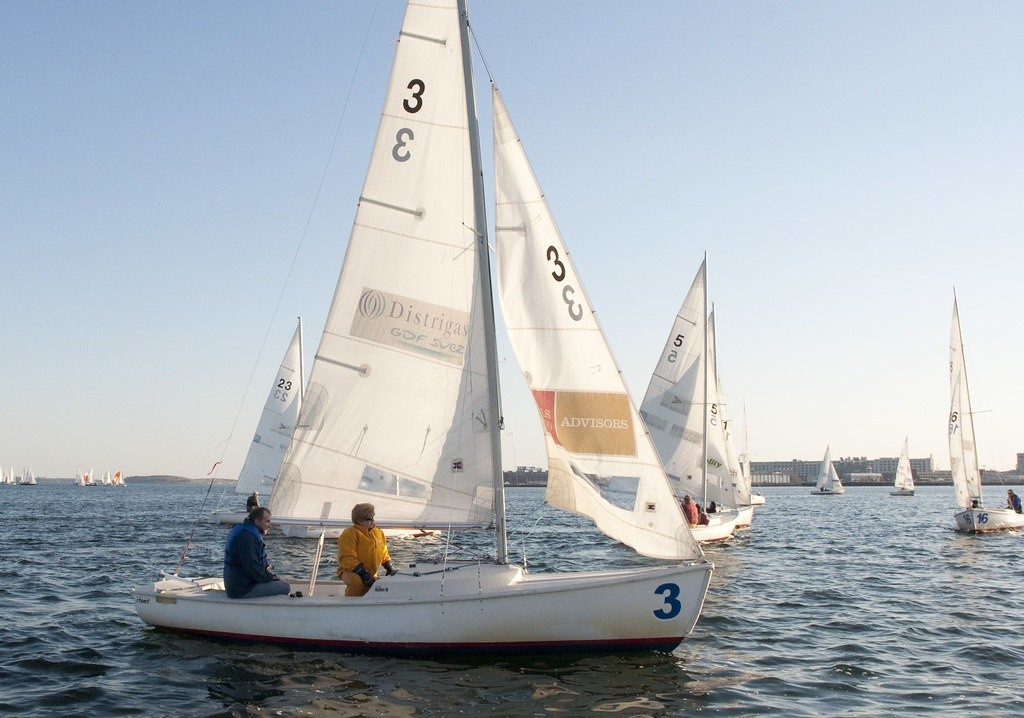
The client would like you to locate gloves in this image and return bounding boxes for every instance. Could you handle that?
[352,564,375,588]
[382,561,398,575]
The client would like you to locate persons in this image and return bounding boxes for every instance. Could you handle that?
[820,486,823,491]
[682,495,708,526]
[706,501,716,513]
[1007,489,1022,513]
[223,507,293,598]
[247,491,260,512]
[970,500,981,508]
[338,503,395,597]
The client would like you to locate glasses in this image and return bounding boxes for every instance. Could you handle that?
[366,517,373,521]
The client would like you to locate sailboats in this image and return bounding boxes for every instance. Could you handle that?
[274,521,444,540]
[740,402,766,505]
[205,314,305,524]
[809,444,846,495]
[136,0,715,657]
[0,466,37,485]
[72,466,124,486]
[637,250,739,543]
[707,299,755,528]
[947,286,1024,532]
[888,435,916,496]
